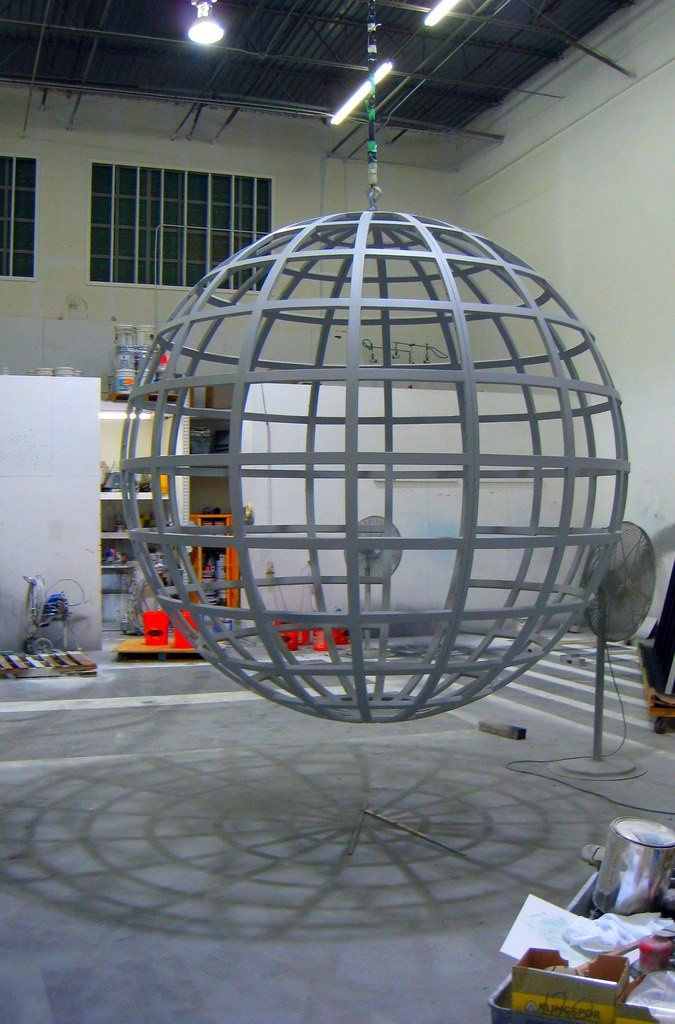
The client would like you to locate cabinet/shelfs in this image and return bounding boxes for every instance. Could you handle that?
[101,399,191,626]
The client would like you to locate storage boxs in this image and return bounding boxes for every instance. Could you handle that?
[487,871,675,1024]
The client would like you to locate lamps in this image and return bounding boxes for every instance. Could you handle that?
[330,60,393,126]
[188,0,224,44]
[422,0,459,27]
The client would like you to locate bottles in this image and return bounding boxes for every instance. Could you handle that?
[629,937,675,1005]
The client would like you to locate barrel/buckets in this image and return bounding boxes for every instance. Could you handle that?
[273,621,349,652]
[592,817,675,916]
[173,610,199,649]
[142,611,170,646]
